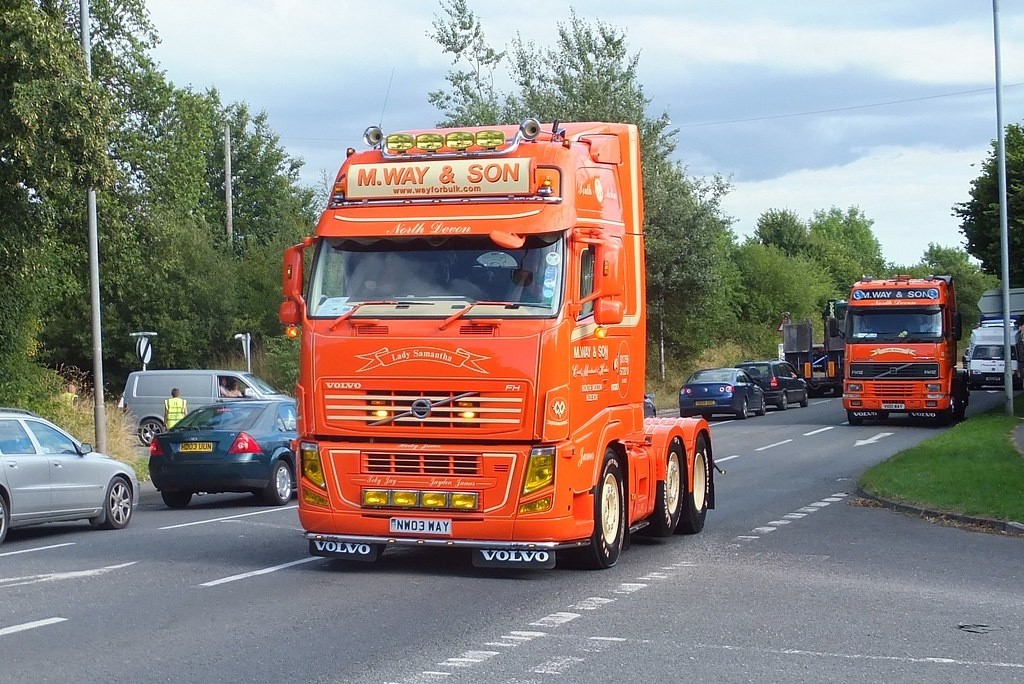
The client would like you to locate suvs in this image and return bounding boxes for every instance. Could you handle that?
[735,357,809,411]
[0,406,141,546]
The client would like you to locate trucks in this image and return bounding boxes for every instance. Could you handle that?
[784,345,843,398]
[979,315,1023,328]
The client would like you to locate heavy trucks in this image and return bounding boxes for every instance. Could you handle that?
[280,118,726,569]
[823,273,970,427]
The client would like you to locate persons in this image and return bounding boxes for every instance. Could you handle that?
[163,388,187,433]
[62,384,79,410]
[219,376,244,398]
[1015,319,1024,382]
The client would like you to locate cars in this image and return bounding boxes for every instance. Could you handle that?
[643,395,656,419]
[679,367,766,421]
[149,398,298,508]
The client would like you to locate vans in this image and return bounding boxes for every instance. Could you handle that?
[124,369,297,447]
[962,325,1019,392]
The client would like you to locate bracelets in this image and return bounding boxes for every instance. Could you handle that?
[163,425,165,427]
